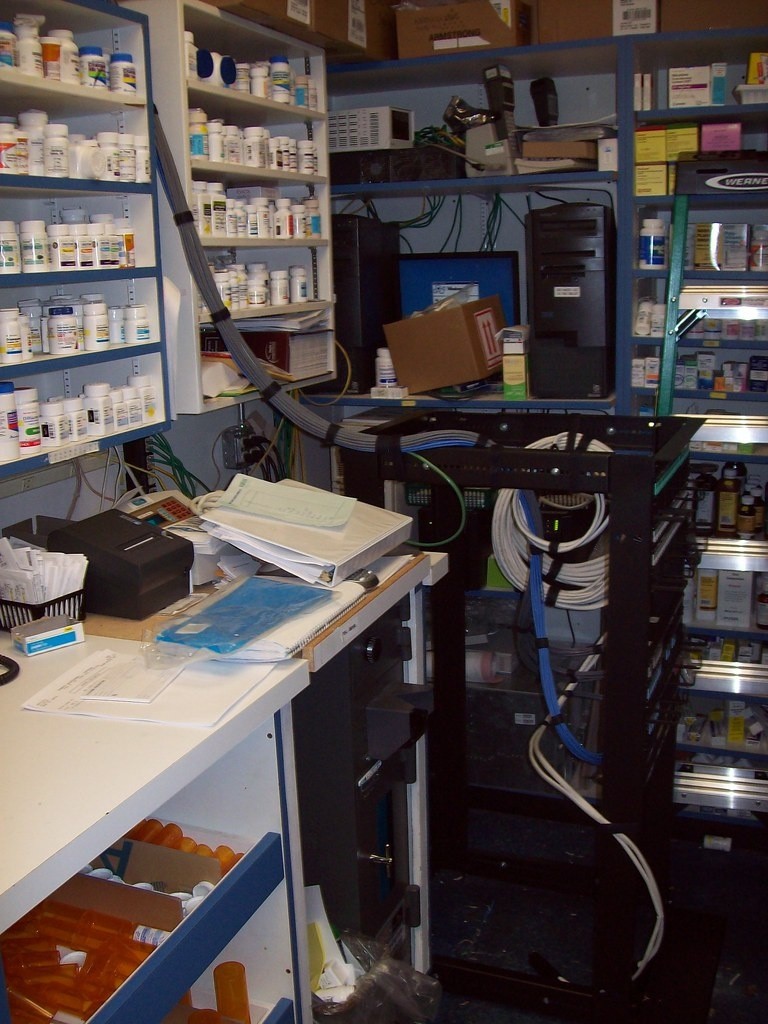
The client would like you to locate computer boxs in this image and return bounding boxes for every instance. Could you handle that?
[524,203,615,400]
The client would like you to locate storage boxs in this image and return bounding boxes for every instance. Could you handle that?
[10,613,86,656]
[210,0,768,397]
[0,588,88,633]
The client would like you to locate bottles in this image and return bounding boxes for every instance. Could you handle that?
[635,303,651,334]
[375,348,397,387]
[651,305,666,337]
[0,29,322,461]
[691,468,765,540]
[639,218,665,271]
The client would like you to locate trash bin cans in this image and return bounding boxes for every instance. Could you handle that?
[311,925,395,1024]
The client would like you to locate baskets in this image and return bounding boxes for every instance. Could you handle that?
[0,588,87,634]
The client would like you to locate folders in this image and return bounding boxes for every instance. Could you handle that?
[198,478,414,588]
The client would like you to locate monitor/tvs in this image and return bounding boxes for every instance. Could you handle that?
[394,250,522,328]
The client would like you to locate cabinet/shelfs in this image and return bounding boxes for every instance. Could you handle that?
[324,22,768,829]
[338,407,725,1024]
[112,0,339,415]
[0,0,174,482]
[0,550,450,1024]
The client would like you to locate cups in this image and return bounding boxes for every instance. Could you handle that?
[0,819,246,1024]
[213,960,251,1024]
